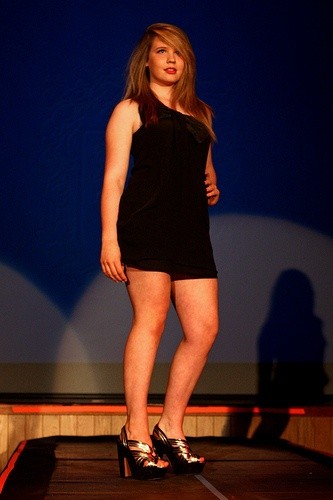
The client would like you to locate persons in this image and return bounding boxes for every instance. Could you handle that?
[97,22,225,480]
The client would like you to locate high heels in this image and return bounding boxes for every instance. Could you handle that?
[152,423,206,476]
[116,425,166,479]
